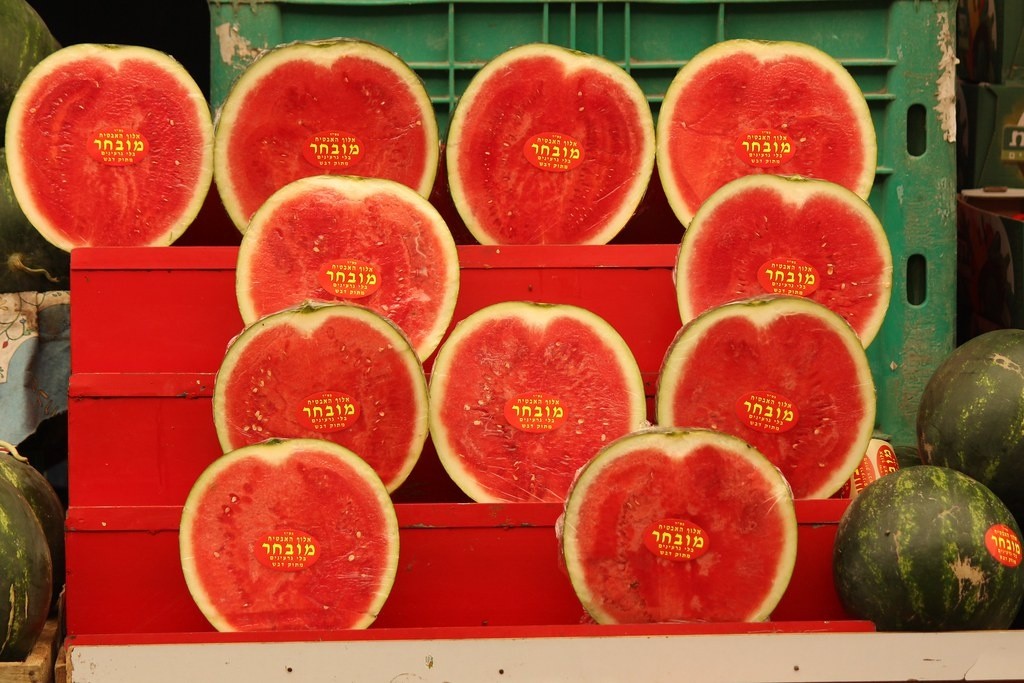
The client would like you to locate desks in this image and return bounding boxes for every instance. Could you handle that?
[67,630,1024,683]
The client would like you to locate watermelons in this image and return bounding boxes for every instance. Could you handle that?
[0,0,1024,662]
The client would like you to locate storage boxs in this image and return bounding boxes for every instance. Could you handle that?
[209,0,959,446]
